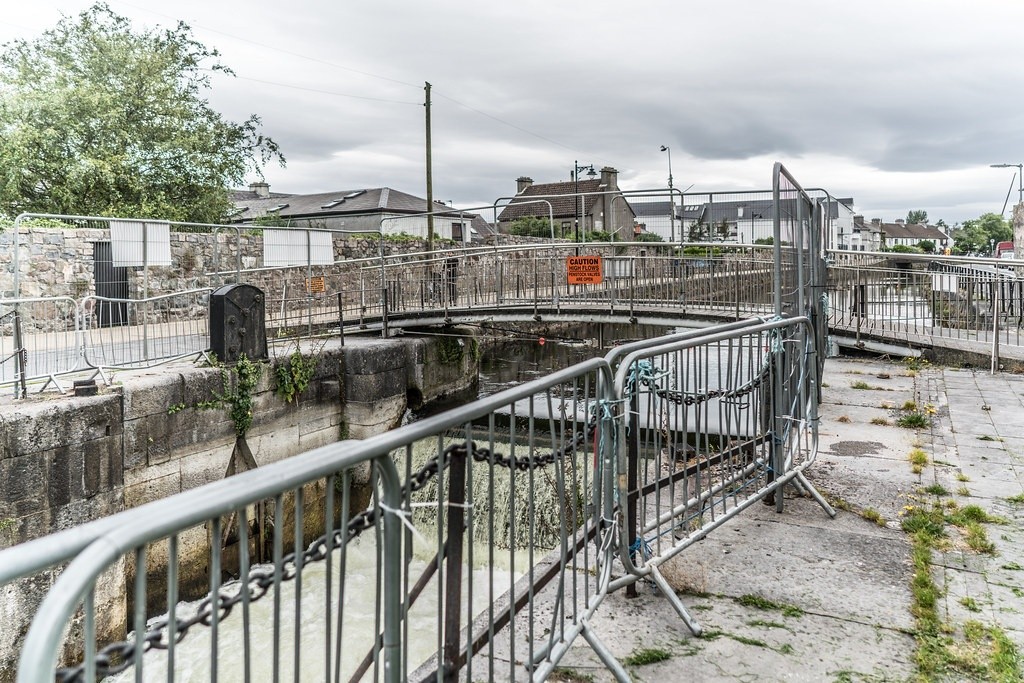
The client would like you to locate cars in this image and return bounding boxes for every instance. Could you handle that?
[997,252,1015,272]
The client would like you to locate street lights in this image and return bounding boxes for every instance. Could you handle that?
[575,160,597,256]
[660,145,675,257]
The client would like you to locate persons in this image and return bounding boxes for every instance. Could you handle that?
[441,251,459,306]
